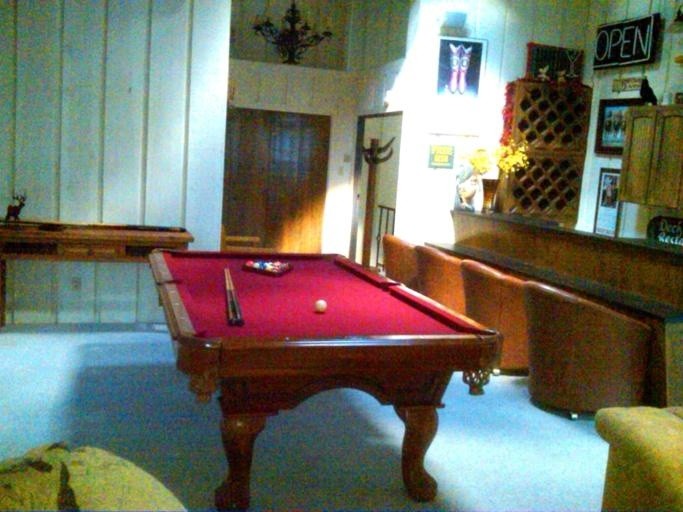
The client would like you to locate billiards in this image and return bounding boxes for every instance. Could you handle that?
[246,260,293,274]
[315,299,328,312]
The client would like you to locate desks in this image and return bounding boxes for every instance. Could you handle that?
[0,218,193,327]
[149,248,504,511]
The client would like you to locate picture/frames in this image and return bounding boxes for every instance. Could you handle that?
[593,168,623,238]
[594,98,656,155]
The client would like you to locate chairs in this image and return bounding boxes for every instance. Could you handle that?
[415,238,464,316]
[521,277,656,417]
[379,233,414,289]
[458,257,528,376]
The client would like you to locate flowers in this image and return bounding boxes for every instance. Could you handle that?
[470,139,529,181]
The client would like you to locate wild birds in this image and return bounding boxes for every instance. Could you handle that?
[639,77,658,105]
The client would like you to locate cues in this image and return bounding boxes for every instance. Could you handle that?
[224,263,235,324]
[0,216,186,234]
[228,268,244,324]
